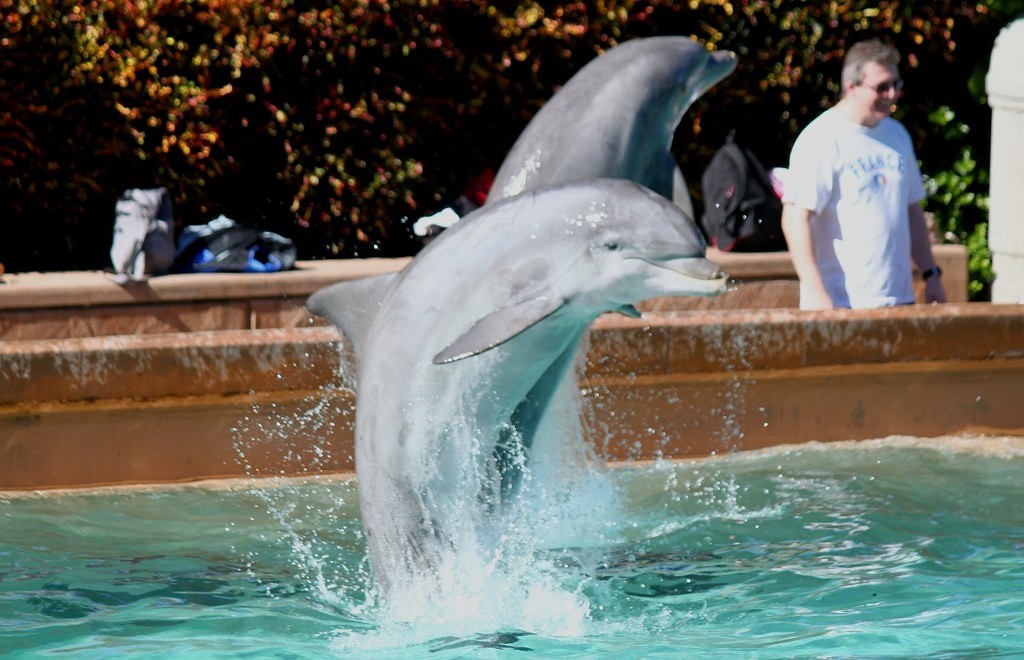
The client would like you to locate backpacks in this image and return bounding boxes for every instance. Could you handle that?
[110,187,174,285]
[701,129,783,252]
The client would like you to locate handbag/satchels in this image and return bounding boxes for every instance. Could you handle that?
[175,214,295,272]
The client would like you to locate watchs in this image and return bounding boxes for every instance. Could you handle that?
[922,266,943,280]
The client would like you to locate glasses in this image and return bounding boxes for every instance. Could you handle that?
[855,79,904,93]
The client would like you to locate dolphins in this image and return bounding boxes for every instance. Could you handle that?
[302,35,740,612]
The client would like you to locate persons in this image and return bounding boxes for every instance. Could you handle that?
[780,39,947,309]
